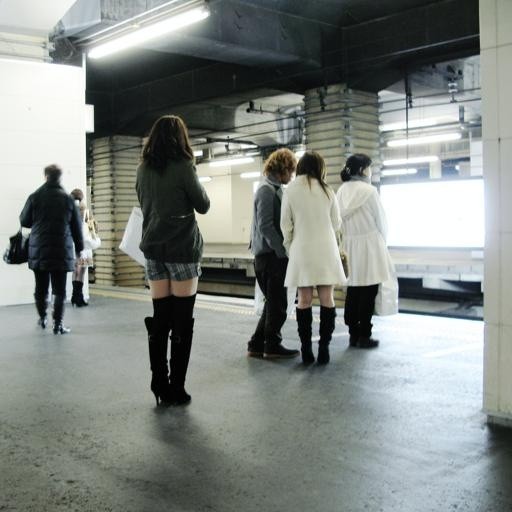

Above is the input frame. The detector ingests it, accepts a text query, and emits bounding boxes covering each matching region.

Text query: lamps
[383,154,439,167]
[378,113,461,133]
[240,170,261,179]
[385,130,463,148]
[381,168,418,177]
[209,154,255,168]
[198,176,212,184]
[85,2,213,62]
[192,149,205,158]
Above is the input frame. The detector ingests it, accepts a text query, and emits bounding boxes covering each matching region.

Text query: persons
[247,149,300,360]
[19,164,84,336]
[70,189,93,309]
[334,155,394,350]
[135,114,209,406]
[280,153,346,367]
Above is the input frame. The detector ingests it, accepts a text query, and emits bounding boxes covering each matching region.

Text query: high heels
[52,319,70,336]
[38,318,48,329]
[71,280,88,308]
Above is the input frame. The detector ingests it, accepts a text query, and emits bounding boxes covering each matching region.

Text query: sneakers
[264,345,299,358]
[359,339,379,348]
[349,336,359,347]
[247,343,263,357]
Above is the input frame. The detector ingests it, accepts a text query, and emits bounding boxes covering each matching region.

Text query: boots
[317,304,337,364]
[295,306,315,365]
[144,317,170,405]
[167,317,195,407]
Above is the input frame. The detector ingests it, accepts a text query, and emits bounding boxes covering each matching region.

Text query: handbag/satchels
[81,209,101,249]
[3,224,29,265]
[337,233,348,278]
[117,206,146,267]
[374,247,399,316]
[253,274,297,318]
